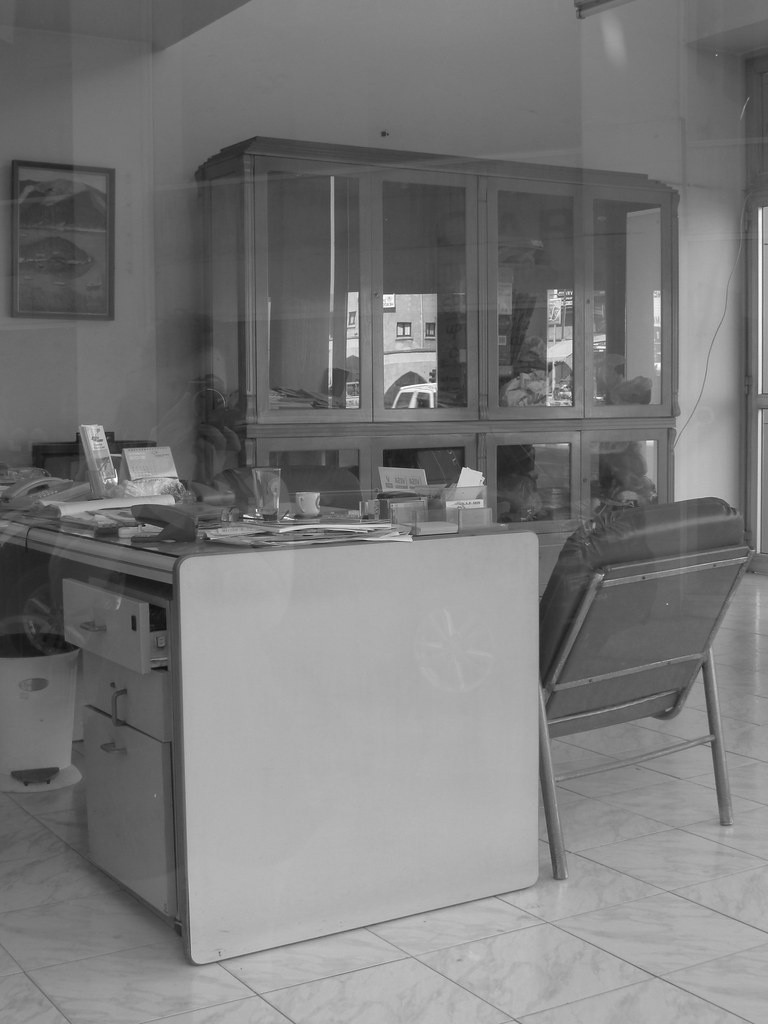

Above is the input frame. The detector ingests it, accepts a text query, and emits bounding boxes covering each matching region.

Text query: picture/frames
[11,159,115,320]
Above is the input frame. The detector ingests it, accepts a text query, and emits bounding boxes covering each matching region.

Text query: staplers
[131,505,198,542]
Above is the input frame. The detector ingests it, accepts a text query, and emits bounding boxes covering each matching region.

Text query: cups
[296,492,321,518]
[359,501,368,519]
[253,467,280,520]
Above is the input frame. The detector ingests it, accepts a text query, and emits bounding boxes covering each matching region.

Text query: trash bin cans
[0,633,82,793]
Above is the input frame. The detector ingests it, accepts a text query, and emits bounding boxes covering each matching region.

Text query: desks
[0,459,543,964]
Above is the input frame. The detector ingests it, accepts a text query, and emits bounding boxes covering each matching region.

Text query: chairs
[535,496,755,882]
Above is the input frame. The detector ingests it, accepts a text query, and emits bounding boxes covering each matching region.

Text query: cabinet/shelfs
[475,156,680,424]
[474,424,676,600]
[191,135,478,428]
[219,429,480,541]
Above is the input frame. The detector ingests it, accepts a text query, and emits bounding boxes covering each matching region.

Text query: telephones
[1,476,92,509]
